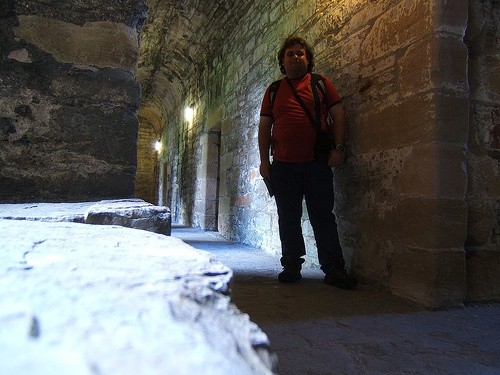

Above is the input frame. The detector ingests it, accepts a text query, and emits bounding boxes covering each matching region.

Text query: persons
[258,36,360,291]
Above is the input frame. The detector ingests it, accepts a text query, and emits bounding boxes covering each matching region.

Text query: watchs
[332,142,346,153]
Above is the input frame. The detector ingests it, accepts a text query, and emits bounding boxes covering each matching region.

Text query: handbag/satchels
[313,129,333,161]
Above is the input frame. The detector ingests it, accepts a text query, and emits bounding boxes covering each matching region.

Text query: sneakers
[324,269,357,289]
[279,268,301,282]
